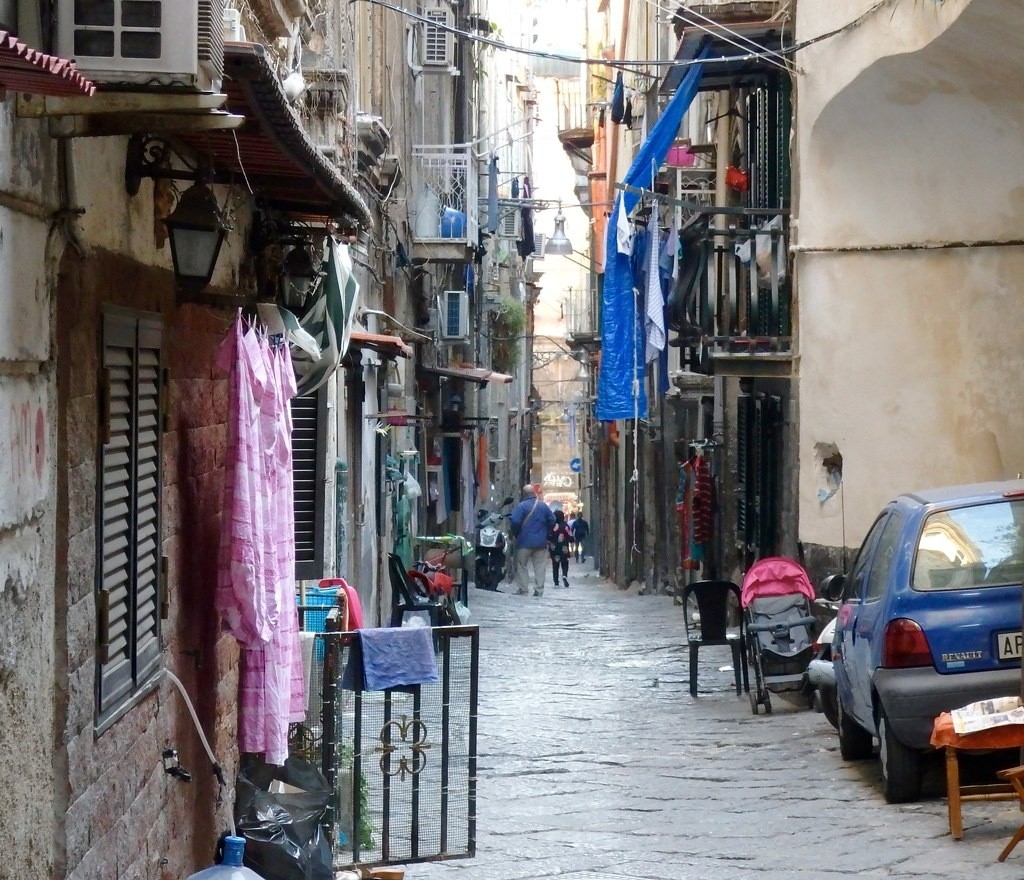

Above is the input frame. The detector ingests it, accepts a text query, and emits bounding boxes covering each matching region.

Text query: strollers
[737,556,826,715]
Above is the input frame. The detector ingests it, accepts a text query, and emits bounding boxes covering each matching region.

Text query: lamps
[248,227,322,296]
[127,133,272,289]
[450,395,461,411]
[478,196,573,257]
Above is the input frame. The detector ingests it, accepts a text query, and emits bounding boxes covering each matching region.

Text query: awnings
[125,42,376,239]
[656,20,792,95]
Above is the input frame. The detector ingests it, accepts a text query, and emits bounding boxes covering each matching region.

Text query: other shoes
[555,582,559,586]
[562,575,569,587]
[582,558,585,563]
[576,558,579,563]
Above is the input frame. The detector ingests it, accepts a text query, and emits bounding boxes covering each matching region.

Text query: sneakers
[512,588,528,596]
[532,589,543,597]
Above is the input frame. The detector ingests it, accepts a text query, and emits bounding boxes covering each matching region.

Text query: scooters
[475,497,516,590]
[807,598,840,729]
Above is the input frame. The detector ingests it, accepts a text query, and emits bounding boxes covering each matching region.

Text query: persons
[509,485,557,597]
[566,512,589,563]
[548,510,575,586]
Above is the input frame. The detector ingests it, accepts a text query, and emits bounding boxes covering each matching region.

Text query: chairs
[930,695,1024,841]
[386,553,449,654]
[998,765,1024,863]
[682,580,748,697]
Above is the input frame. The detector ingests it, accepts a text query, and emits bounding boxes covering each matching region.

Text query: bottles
[186,836,268,880]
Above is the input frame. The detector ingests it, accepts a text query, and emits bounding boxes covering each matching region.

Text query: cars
[821,478,1024,803]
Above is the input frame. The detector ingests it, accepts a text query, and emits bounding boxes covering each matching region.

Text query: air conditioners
[442,290,470,340]
[52,0,226,92]
[530,233,546,260]
[500,207,522,237]
[421,7,455,67]
